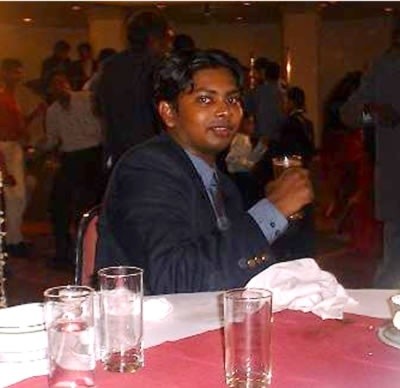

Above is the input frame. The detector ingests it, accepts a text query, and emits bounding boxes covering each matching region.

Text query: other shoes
[6,242,28,255]
[50,255,74,268]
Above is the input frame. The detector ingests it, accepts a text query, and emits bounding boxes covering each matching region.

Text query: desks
[1,288,400,388]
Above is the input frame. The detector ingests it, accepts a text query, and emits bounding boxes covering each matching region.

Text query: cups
[220,285,274,388]
[97,265,145,374]
[42,283,97,388]
[273,151,305,220]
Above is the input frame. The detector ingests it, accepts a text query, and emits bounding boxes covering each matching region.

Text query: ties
[209,172,227,229]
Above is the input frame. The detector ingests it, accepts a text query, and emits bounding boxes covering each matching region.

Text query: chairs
[0,142,26,249]
[73,208,99,292]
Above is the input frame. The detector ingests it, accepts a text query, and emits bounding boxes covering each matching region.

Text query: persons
[0,58,34,257]
[31,72,105,270]
[169,35,314,259]
[319,70,380,256]
[95,49,315,297]
[38,40,117,101]
[91,10,175,185]
[339,3,400,257]
